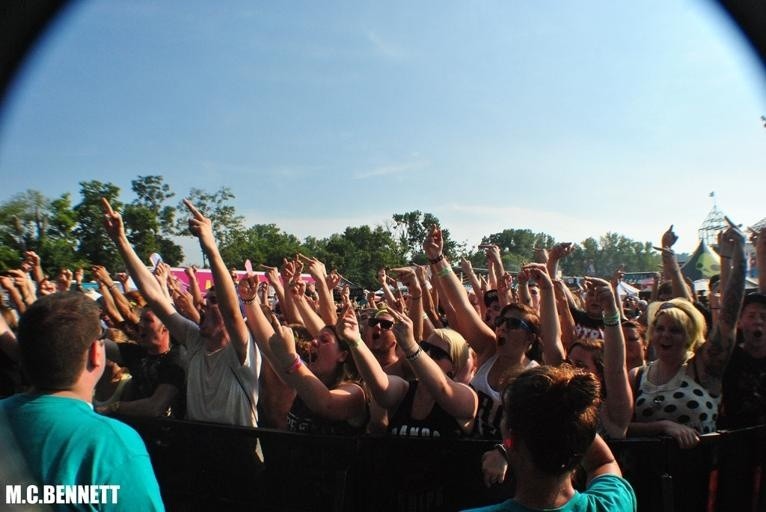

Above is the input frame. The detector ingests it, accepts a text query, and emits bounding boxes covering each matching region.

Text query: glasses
[88,327,109,352]
[367,318,394,329]
[419,340,454,363]
[494,315,533,334]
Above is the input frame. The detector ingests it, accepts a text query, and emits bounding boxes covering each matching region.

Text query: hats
[484,289,501,308]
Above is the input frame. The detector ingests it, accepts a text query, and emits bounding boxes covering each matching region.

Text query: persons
[2,192,766,511]
